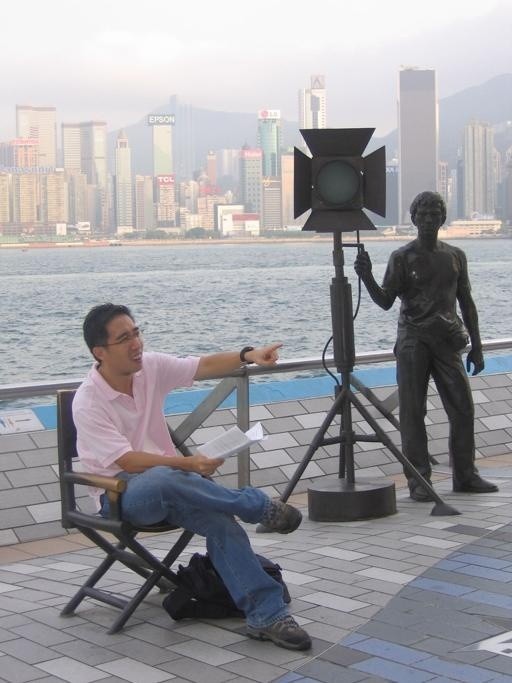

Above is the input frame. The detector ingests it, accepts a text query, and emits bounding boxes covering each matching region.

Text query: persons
[69,301,313,652]
[354,191,499,502]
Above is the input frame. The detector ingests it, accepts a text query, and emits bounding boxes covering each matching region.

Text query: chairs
[56,390,196,636]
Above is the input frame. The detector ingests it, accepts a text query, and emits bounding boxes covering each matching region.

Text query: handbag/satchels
[164,551,292,620]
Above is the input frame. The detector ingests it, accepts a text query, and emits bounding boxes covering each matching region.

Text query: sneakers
[245,615,311,651]
[259,499,303,535]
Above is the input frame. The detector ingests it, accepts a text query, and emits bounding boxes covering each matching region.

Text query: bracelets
[239,347,255,366]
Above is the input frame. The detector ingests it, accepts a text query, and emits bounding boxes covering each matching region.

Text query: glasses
[90,328,145,353]
